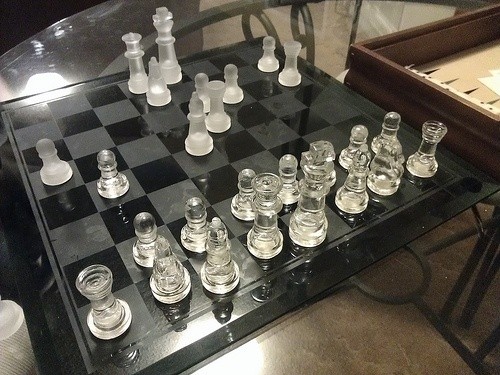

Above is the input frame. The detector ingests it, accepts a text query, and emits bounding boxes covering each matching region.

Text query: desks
[0,0,499,374]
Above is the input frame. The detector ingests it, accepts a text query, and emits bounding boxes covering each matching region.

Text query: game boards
[0,35,500,375]
